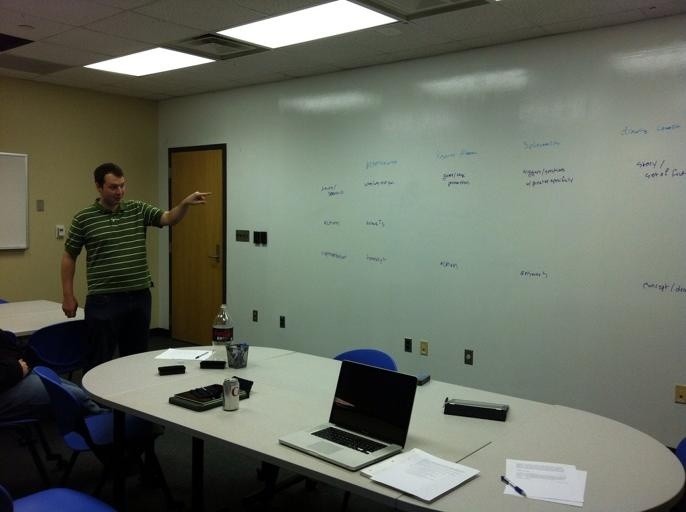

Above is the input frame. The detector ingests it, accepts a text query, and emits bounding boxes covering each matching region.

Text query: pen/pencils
[195,352,208,359]
[227,342,248,368]
[501,475,526,497]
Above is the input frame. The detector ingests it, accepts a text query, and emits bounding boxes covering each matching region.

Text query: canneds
[223,378,239,411]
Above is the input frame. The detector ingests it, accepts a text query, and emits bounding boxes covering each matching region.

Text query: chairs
[674,437,686,467]
[34,366,163,484]
[3,418,53,488]
[331,350,398,371]
[0,474,109,512]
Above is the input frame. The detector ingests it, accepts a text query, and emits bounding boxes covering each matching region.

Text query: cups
[226,343,248,369]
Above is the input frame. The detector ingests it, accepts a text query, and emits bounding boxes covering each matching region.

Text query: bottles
[212,304,234,368]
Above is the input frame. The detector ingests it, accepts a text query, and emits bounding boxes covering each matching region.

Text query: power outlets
[236,230,249,241]
[55,224,65,239]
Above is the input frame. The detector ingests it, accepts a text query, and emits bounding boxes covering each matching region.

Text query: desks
[1,300,86,340]
[81,343,686,511]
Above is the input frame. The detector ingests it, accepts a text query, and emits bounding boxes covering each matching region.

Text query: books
[360,447,480,503]
[169,383,249,412]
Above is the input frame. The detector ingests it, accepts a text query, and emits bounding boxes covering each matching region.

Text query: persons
[0,330,101,415]
[62,163,213,373]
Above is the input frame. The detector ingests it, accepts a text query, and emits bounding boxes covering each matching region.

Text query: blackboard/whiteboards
[0,152,30,250]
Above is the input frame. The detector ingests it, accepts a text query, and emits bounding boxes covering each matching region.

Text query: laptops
[278,359,418,472]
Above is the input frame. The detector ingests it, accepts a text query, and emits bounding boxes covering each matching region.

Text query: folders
[443,396,510,422]
